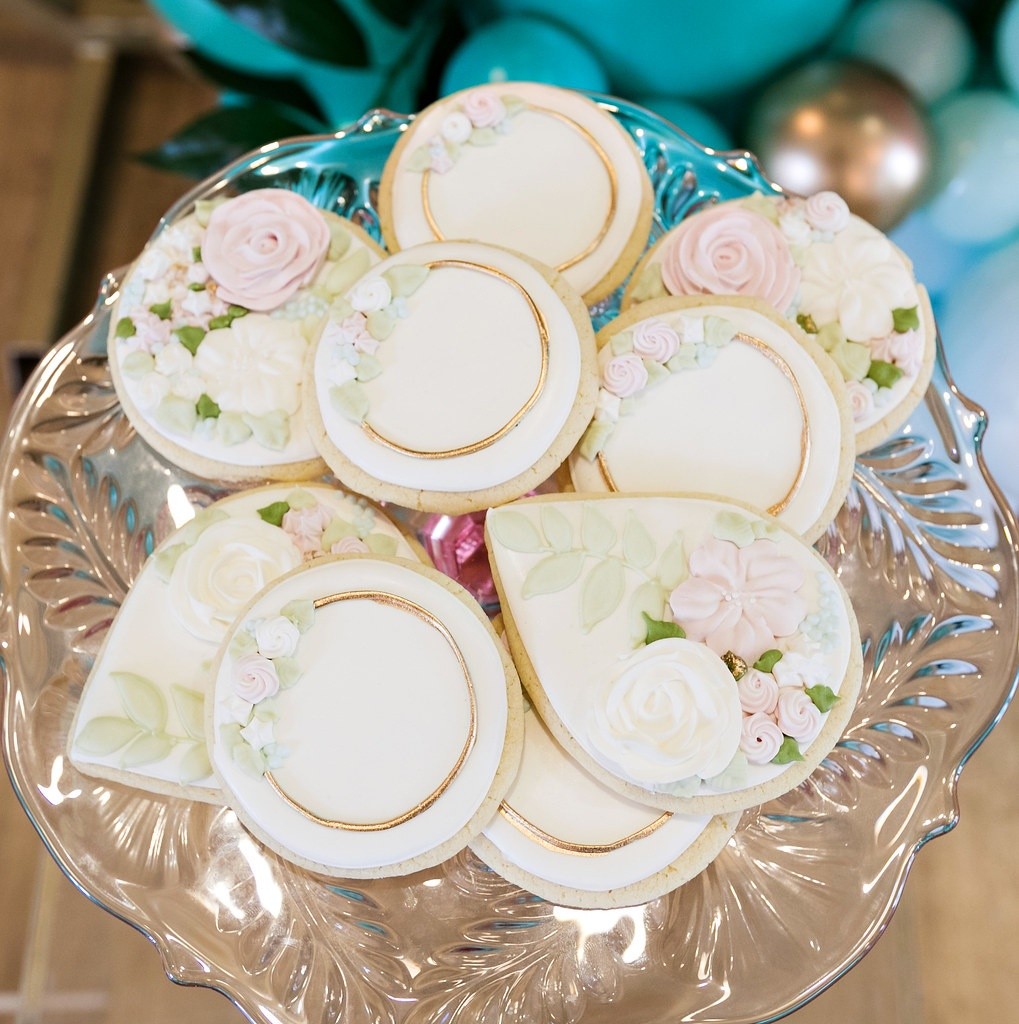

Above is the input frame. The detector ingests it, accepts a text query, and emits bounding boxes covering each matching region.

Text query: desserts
[62,80,939,917]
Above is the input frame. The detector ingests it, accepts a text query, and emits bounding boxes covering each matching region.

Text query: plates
[0,91,1019,1023]
